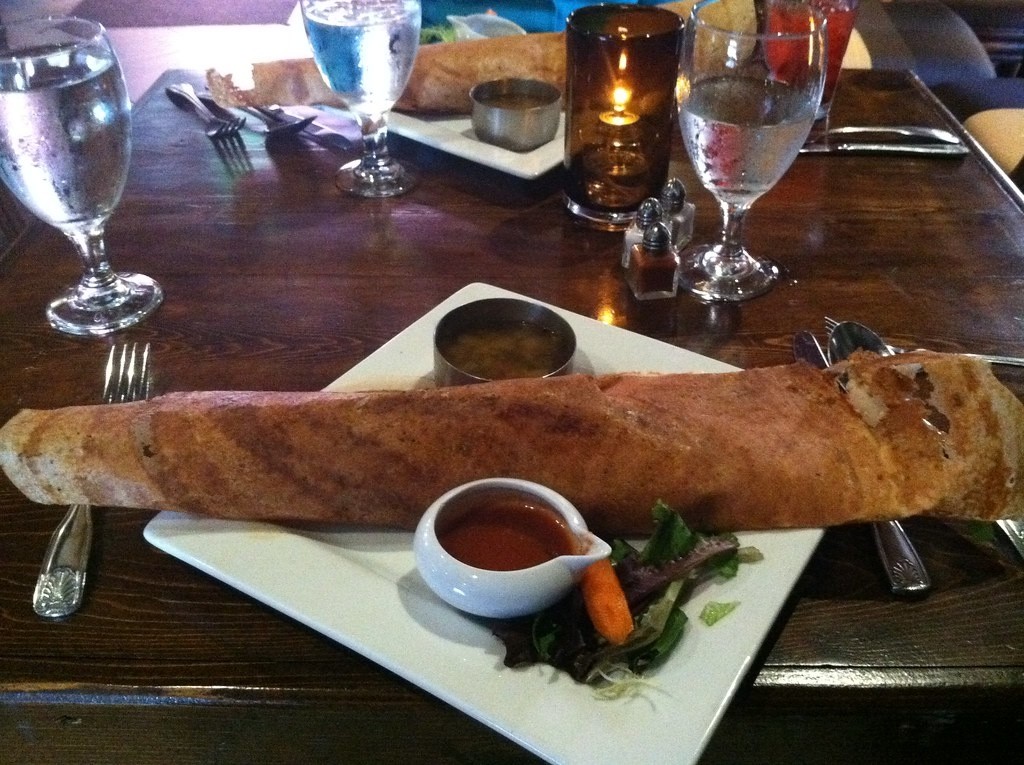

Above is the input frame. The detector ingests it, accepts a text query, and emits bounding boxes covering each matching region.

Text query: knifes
[793,142,971,154]
[794,327,931,597]
[258,101,353,152]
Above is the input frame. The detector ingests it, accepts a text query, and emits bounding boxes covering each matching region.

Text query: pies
[206,0,758,113]
[0,351,1024,529]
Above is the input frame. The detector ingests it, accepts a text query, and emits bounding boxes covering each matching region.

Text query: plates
[337,77,594,180]
[142,282,825,765]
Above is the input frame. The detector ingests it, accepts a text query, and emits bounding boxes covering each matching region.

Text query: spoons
[235,94,317,138]
[827,320,1024,572]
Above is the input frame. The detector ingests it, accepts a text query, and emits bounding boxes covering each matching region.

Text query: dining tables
[0,68,1024,765]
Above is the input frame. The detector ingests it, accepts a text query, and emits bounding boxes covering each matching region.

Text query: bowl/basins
[429,297,578,388]
[468,80,563,151]
[414,475,613,616]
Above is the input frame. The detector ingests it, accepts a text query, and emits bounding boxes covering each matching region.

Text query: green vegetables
[490,498,762,678]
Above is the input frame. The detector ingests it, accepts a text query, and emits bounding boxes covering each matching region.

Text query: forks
[820,315,1023,377]
[165,81,247,138]
[31,341,156,616]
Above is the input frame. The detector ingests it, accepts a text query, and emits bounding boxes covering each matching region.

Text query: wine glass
[0,13,166,337]
[300,1,422,196]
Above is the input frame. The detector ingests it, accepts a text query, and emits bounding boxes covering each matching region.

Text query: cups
[673,1,829,300]
[763,1,861,120]
[560,4,685,223]
